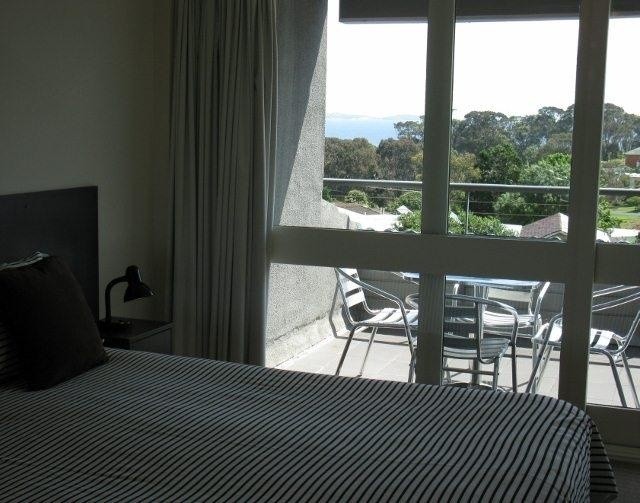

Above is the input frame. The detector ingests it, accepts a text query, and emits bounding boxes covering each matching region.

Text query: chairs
[329,266,418,377]
[405,292,518,393]
[526,285,639,408]
[451,277,552,393]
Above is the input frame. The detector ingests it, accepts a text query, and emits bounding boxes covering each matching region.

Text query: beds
[0,185,618,503]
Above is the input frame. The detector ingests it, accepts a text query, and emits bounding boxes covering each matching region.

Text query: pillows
[0,251,51,383]
[0,254,109,391]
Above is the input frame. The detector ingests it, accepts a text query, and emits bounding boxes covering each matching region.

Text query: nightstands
[99,317,175,355]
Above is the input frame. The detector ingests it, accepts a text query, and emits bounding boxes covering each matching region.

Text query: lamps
[100,264,161,331]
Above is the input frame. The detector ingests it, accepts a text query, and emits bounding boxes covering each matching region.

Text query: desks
[404,274,542,392]
